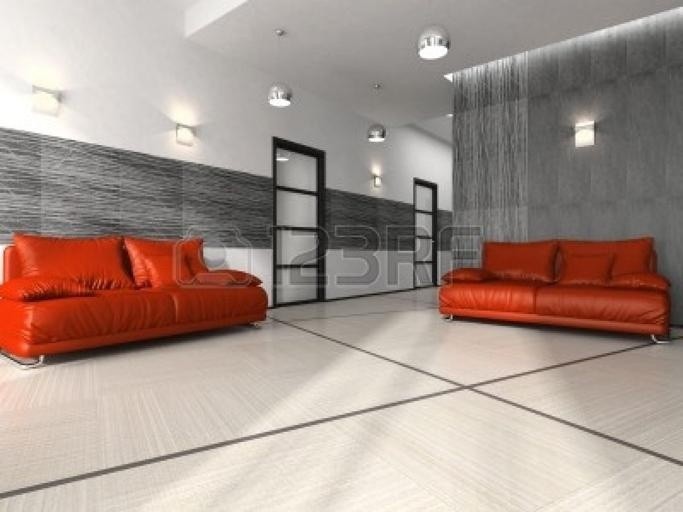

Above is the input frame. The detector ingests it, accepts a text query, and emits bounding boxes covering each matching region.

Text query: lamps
[364,123,386,144]
[266,81,292,108]
[413,23,450,60]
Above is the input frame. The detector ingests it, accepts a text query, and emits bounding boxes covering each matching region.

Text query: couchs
[0,231,267,368]
[437,236,673,344]
